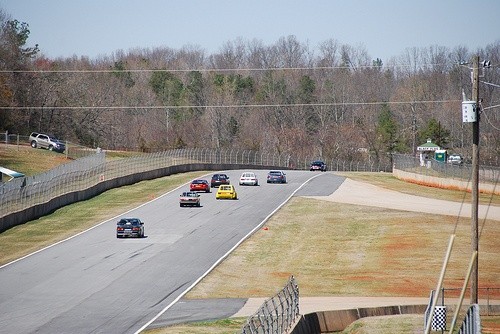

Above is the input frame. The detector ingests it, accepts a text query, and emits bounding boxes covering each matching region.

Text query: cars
[266,170,287,184]
[309,160,327,171]
[116,218,145,238]
[216,185,238,200]
[179,191,201,207]
[189,179,210,193]
[239,172,259,186]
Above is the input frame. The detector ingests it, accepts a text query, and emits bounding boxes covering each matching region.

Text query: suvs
[446,155,464,168]
[28,131,66,153]
[211,173,231,188]
